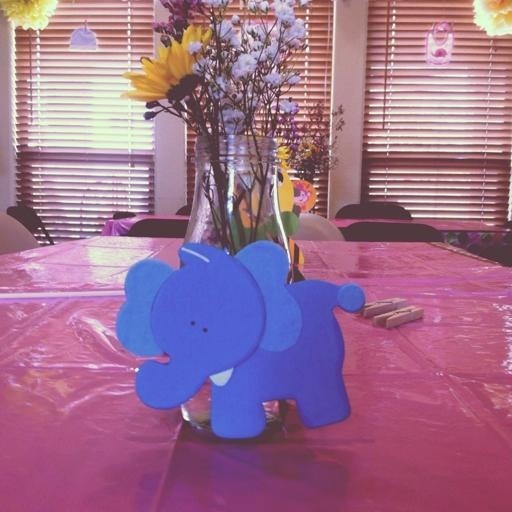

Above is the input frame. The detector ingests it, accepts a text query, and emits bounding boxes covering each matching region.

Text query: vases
[180,136,292,287]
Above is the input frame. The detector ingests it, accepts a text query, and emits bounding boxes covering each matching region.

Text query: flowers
[0,0,59,32]
[472,1,512,37]
[120,1,346,256]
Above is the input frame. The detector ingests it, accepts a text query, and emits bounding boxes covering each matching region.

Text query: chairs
[346,221,442,243]
[335,203,412,222]
[128,219,191,236]
[6,202,54,244]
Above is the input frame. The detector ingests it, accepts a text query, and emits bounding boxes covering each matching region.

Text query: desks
[104,214,511,237]
[1,237,511,511]
